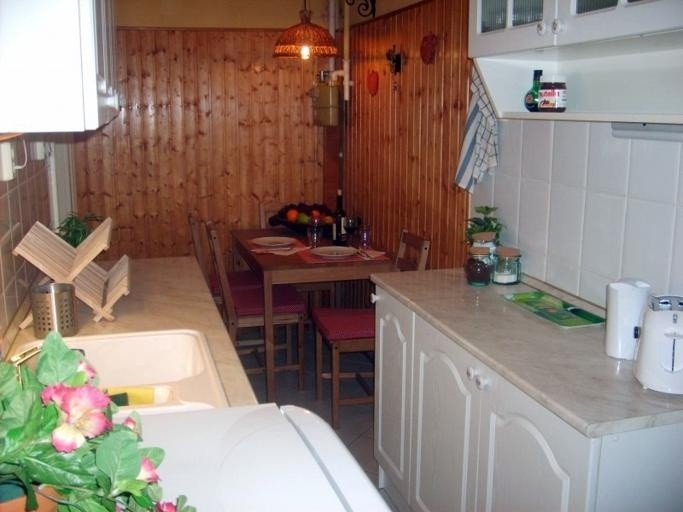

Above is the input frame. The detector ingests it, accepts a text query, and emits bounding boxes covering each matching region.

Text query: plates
[501,290,606,330]
[251,236,296,250]
[309,245,358,260]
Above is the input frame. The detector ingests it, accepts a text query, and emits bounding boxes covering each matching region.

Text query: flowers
[0,331,198,512]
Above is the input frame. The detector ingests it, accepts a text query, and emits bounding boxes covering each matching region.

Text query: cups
[359,224,371,250]
[343,216,358,244]
[307,217,321,247]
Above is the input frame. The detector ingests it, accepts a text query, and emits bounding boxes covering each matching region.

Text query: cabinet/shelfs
[0,0,119,133]
[0,255,259,408]
[370,268,683,509]
[466,0,683,57]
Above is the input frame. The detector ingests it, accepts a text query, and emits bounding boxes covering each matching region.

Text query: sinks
[5,329,230,423]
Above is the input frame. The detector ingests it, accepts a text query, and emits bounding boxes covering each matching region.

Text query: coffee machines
[604,278,652,360]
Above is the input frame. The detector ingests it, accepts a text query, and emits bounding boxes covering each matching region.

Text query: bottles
[524,70,542,112]
[466,248,491,287]
[538,75,567,113]
[492,247,521,284]
[332,189,346,243]
[471,232,496,265]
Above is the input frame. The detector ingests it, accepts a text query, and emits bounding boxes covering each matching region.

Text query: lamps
[273,2,338,57]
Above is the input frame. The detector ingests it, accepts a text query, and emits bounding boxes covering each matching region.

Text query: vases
[0,480,65,512]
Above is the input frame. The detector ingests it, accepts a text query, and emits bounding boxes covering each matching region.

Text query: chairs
[314,229,431,429]
[205,219,305,394]
[188,212,264,355]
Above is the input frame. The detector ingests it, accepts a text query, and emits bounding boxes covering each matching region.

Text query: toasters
[632,293,683,395]
